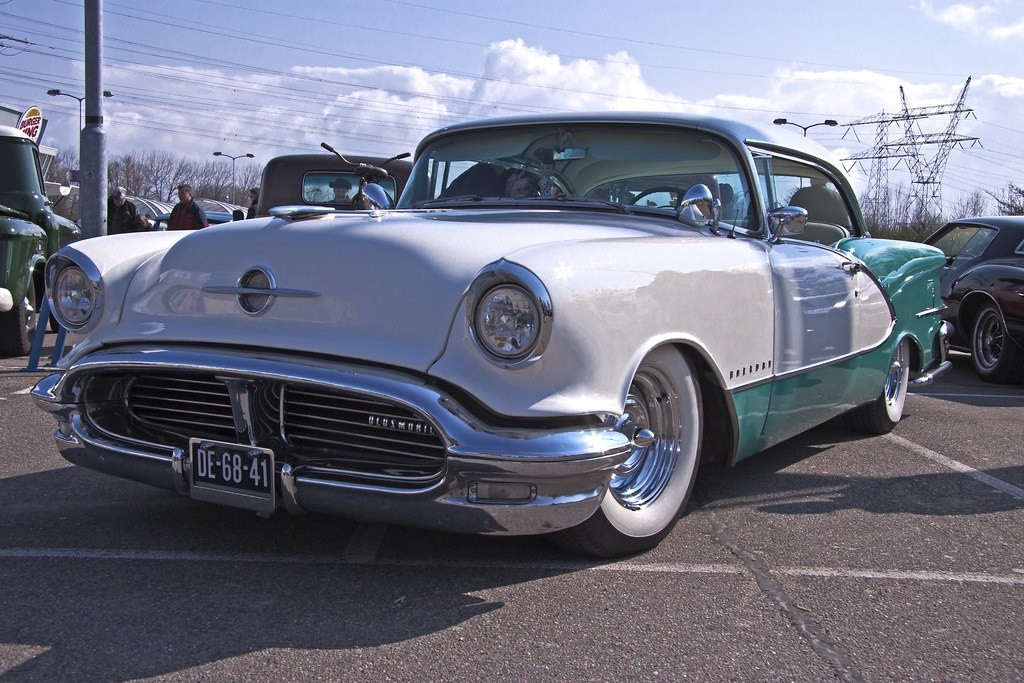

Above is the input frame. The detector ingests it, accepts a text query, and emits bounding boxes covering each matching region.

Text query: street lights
[48,87,115,132]
[772,117,838,188]
[211,151,255,205]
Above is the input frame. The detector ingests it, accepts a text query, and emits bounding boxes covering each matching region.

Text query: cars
[920,216,1024,383]
[194,198,250,222]
[253,154,431,219]
[126,195,176,232]
[0,123,60,357]
[28,106,947,557]
[53,211,82,249]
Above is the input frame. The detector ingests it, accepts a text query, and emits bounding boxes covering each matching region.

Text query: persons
[434,163,544,203]
[246,187,261,219]
[788,176,852,228]
[107,187,140,236]
[328,178,353,203]
[167,183,209,230]
[139,214,153,232]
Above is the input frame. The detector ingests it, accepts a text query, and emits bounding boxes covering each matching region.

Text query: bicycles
[320,140,410,210]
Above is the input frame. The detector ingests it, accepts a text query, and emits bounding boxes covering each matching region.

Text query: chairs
[719,183,851,246]
